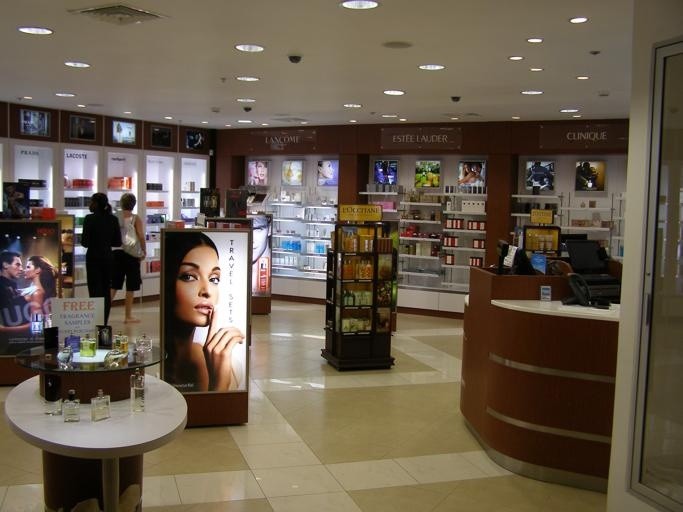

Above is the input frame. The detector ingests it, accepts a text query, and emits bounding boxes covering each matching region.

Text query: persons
[80,192,123,326]
[250,216,271,294]
[0,255,57,336]
[108,192,148,326]
[526,160,553,188]
[0,249,31,327]
[246,161,267,185]
[457,163,475,184]
[4,184,25,217]
[575,161,600,192]
[162,232,245,393]
[315,160,337,185]
[470,162,484,182]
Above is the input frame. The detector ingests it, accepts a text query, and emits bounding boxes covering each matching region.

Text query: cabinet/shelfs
[508,191,625,264]
[17,180,201,291]
[318,221,401,372]
[360,190,487,292]
[262,189,339,280]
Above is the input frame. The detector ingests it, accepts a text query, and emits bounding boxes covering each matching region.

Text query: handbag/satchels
[122,214,145,258]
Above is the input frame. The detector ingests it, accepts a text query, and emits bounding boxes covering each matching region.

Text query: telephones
[561,272,591,306]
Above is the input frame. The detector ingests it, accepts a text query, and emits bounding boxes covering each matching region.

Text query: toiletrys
[90,389,111,421]
[30,312,43,337]
[367,181,484,268]
[337,225,374,333]
[43,330,155,371]
[246,189,267,215]
[271,187,337,275]
[259,263,267,295]
[41,372,61,416]
[63,389,80,422]
[512,189,613,249]
[128,367,145,412]
[45,314,52,329]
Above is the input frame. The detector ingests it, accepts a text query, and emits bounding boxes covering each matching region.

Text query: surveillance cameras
[242,105,252,112]
[286,52,305,63]
[450,95,461,102]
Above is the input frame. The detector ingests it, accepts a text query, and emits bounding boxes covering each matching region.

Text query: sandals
[124,315,141,324]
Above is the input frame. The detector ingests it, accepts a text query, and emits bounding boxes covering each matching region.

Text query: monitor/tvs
[496,244,536,275]
[565,239,609,273]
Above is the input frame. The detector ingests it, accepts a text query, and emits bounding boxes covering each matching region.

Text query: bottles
[338,227,371,309]
[517,231,539,250]
[40,312,153,365]
[306,226,327,237]
[39,369,145,423]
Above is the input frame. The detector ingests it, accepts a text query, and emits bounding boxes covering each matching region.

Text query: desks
[3,341,190,511]
